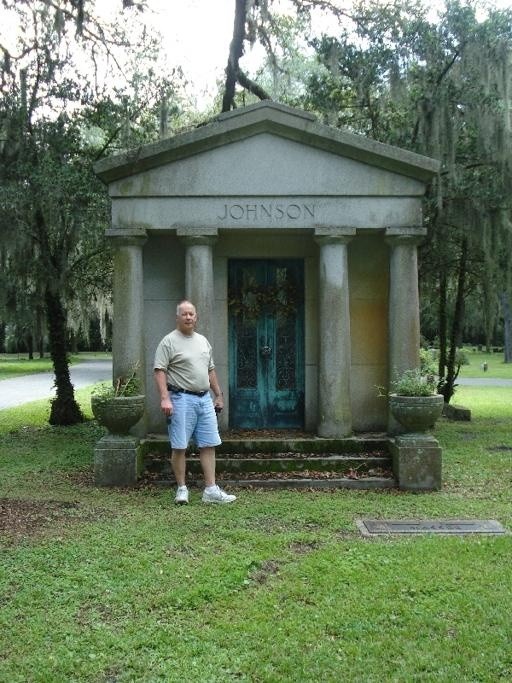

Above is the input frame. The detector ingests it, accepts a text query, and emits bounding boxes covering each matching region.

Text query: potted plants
[372,364,449,440]
[91,359,146,442]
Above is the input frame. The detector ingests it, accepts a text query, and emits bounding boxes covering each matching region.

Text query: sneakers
[174,486,189,504]
[202,485,237,503]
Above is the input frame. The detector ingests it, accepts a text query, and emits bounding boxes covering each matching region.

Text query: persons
[153,300,237,505]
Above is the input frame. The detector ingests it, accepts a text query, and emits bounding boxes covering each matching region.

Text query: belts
[168,384,208,396]
